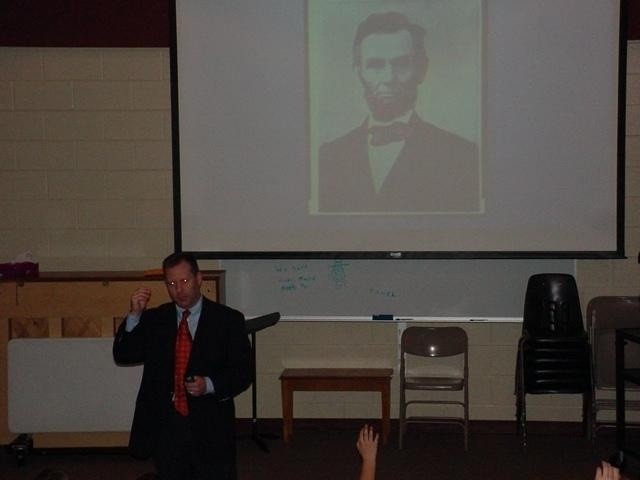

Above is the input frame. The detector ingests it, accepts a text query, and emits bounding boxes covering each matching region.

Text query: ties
[174,311,192,416]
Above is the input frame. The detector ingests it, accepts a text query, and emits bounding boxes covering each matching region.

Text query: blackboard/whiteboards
[216,257,578,325]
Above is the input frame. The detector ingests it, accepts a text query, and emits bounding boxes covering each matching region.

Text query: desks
[279,366,393,446]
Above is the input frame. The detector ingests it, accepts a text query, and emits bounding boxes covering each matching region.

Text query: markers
[469,319,488,321]
[395,318,414,320]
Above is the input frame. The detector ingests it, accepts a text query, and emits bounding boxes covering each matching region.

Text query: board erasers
[371,314,394,321]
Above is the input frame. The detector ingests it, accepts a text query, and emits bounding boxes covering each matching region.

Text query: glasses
[165,275,196,288]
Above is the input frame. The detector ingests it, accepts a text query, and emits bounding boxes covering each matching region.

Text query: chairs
[513,272,591,458]
[587,294,639,439]
[399,324,470,453]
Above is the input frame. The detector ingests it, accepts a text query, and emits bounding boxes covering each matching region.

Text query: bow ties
[369,122,408,145]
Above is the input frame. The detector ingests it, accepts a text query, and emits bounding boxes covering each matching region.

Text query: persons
[594,461,621,480]
[112,252,254,480]
[355,424,380,480]
[317,9,480,216]
